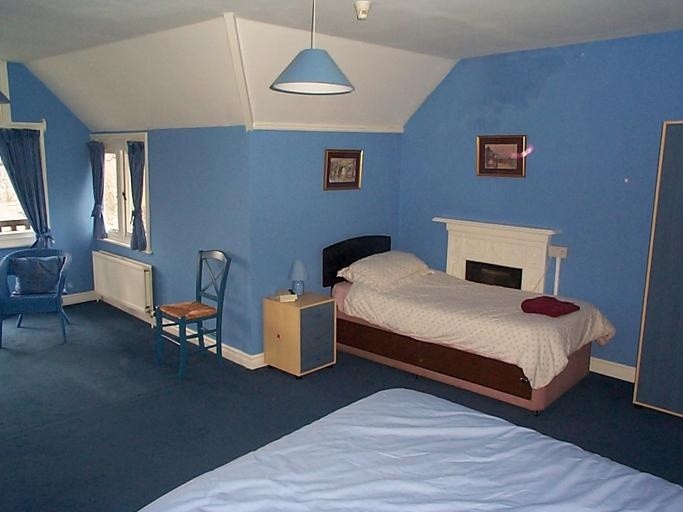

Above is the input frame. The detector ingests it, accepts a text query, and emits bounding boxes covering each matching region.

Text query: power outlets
[548,245,567,259]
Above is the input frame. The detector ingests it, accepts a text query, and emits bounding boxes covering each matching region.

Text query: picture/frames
[475,134,527,178]
[322,149,364,192]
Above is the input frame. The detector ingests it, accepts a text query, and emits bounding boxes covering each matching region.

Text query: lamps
[268,0,355,95]
[289,258,309,296]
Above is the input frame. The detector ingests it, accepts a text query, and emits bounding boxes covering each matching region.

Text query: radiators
[93,250,151,315]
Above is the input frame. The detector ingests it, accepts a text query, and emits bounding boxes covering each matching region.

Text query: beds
[322,234,616,415]
[138,387,682,512]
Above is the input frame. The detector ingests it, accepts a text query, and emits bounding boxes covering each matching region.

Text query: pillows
[11,256,68,295]
[337,250,436,291]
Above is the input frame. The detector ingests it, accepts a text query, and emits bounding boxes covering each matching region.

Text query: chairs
[1,247,73,348]
[157,250,231,384]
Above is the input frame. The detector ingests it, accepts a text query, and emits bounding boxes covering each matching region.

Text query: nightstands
[262,293,337,377]
